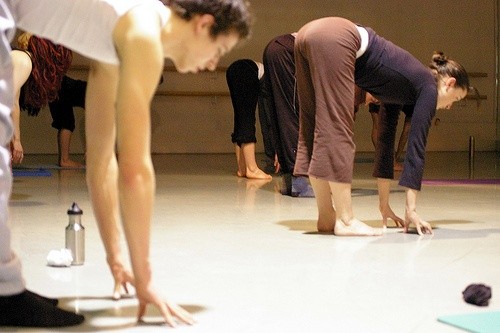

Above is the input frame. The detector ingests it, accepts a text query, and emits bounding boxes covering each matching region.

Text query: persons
[0,0,252,327]
[47,75,164,167]
[262,32,413,197]
[368,102,414,171]
[10,35,73,165]
[13,31,30,50]
[226,59,280,179]
[292,17,470,238]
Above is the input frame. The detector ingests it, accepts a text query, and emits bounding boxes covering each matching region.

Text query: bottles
[65,202,85,266]
